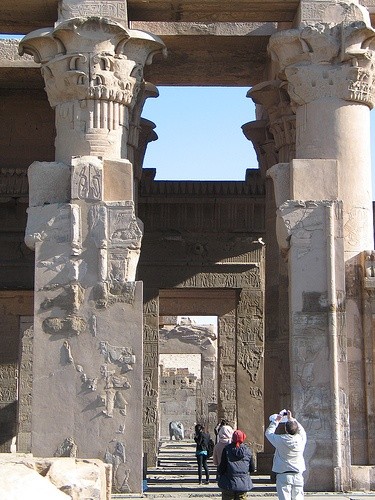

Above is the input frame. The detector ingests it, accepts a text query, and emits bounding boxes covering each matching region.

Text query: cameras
[282,412,288,415]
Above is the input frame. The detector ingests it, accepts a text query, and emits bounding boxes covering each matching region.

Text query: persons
[194,424,210,485]
[217,430,255,500]
[213,420,234,481]
[265,409,307,500]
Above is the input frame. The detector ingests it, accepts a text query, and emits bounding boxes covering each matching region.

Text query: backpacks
[201,432,214,457]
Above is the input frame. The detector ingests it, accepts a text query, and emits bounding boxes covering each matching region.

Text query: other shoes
[204,480,209,484]
[199,481,202,485]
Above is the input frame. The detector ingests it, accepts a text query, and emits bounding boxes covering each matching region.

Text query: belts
[276,471,298,474]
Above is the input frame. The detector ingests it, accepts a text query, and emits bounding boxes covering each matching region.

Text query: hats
[233,430,247,447]
